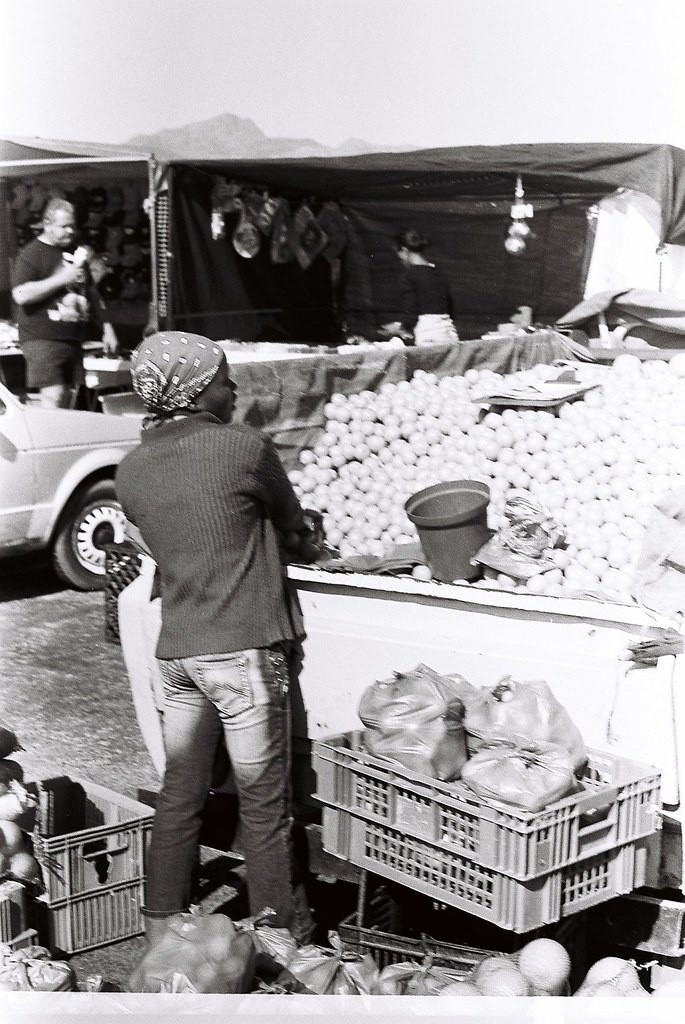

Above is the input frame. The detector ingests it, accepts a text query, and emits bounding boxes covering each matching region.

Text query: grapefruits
[437,938,646,996]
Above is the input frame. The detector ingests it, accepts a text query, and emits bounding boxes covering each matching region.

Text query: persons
[115,330,327,949]
[9,197,120,408]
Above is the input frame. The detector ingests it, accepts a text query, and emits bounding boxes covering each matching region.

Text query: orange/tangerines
[286,369,685,614]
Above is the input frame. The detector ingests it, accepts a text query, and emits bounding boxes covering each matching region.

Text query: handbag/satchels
[2,890,466,996]
[359,663,584,813]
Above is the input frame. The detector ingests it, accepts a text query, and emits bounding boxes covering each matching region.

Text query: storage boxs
[334,886,576,974]
[306,730,665,936]
[18,780,158,958]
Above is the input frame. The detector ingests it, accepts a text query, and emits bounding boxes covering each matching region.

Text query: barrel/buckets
[403,478,491,583]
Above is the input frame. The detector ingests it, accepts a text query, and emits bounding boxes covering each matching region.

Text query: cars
[0,378,148,592]
